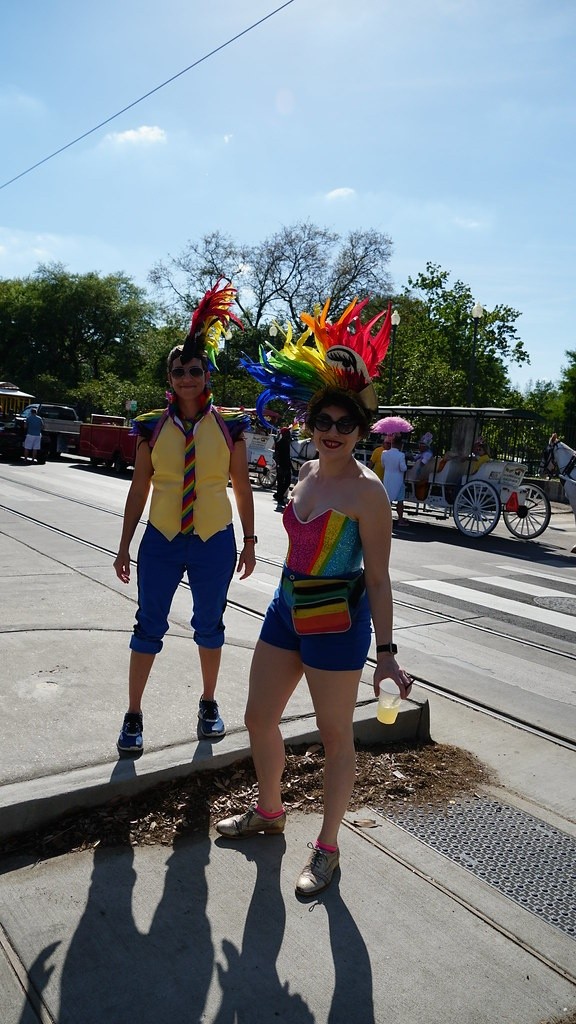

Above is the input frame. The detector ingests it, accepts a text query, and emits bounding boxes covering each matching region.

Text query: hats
[474,437,491,457]
[418,432,433,448]
[280,427,291,433]
[384,435,392,442]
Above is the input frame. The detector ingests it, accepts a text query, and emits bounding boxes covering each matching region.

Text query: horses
[537,433,576,553]
[289,436,319,476]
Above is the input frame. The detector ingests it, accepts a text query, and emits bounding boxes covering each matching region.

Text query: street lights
[466,301,484,409]
[220,328,234,407]
[387,309,402,405]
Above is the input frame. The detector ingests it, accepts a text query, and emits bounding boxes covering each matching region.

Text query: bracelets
[376,644,398,655]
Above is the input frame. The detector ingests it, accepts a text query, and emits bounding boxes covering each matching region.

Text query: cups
[376,678,402,724]
[126,400,137,411]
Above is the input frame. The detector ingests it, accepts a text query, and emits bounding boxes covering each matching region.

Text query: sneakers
[215,802,286,836]
[296,841,340,895]
[116,713,143,750]
[198,695,225,737]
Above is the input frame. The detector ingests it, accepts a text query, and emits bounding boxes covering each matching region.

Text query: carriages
[270,405,552,540]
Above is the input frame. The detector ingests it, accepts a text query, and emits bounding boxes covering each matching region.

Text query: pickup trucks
[15,402,84,432]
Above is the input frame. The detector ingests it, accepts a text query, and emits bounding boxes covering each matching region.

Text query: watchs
[244,536,258,544]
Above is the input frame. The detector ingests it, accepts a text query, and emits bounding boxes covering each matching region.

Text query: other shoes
[397,521,409,526]
[272,493,286,506]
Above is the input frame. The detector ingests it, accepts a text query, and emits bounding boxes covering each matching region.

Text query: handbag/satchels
[292,580,356,633]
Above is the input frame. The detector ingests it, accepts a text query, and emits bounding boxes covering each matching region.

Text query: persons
[381,437,409,526]
[273,426,292,511]
[111,342,258,752]
[214,389,414,899]
[129,413,140,435]
[367,436,392,484]
[470,442,491,476]
[409,440,433,471]
[22,409,45,463]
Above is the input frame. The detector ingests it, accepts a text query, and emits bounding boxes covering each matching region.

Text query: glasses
[171,366,207,379]
[311,411,359,434]
[477,448,484,452]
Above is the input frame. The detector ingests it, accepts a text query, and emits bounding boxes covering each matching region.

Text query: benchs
[429,460,466,483]
[462,461,528,488]
[405,458,436,481]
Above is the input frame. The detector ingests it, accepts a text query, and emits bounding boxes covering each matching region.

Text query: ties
[170,412,205,534]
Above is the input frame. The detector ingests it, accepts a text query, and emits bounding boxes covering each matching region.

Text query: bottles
[125,410,137,427]
